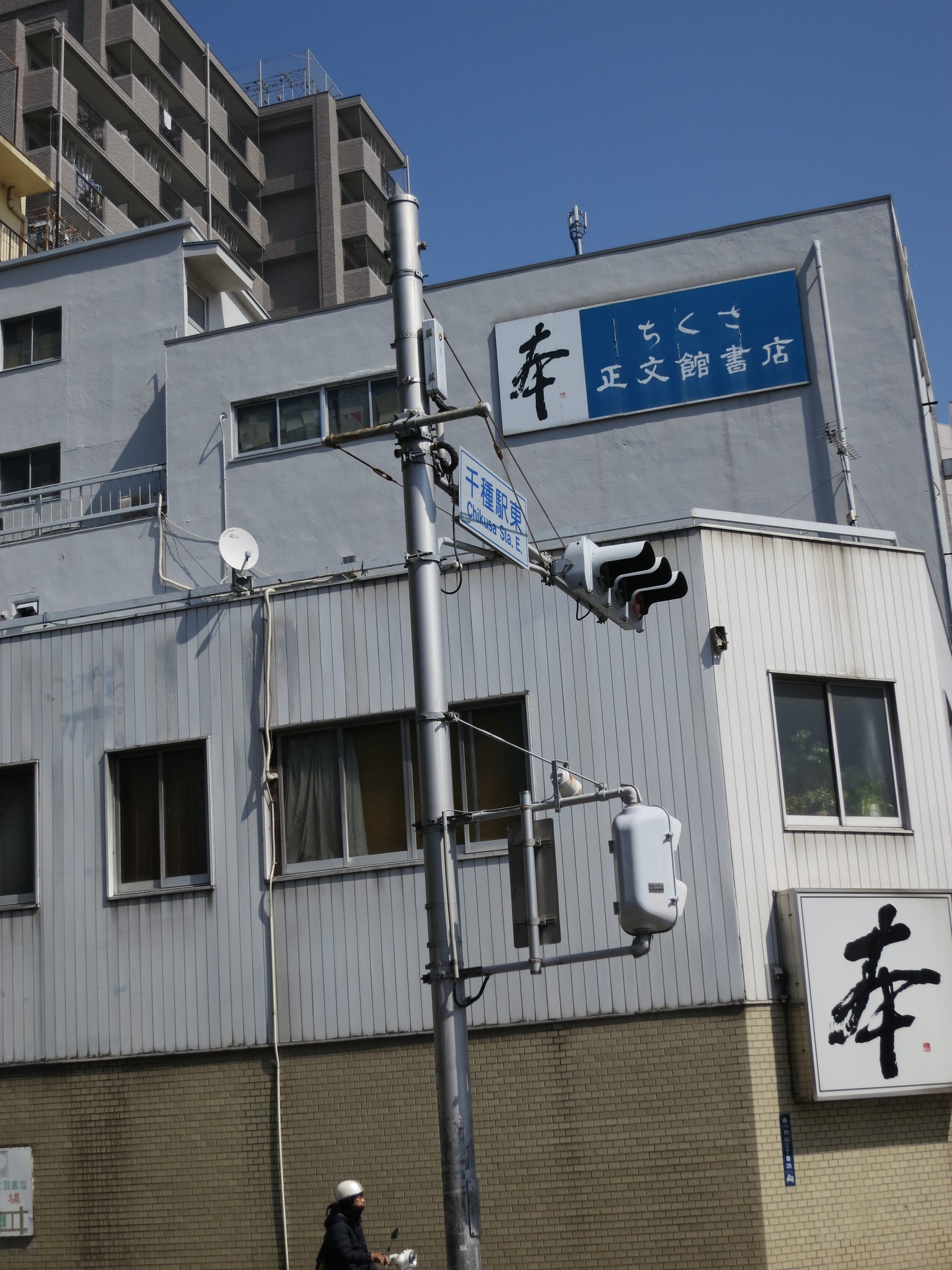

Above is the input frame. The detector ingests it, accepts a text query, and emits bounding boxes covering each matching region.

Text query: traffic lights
[611,804,688,936]
[561,535,689,634]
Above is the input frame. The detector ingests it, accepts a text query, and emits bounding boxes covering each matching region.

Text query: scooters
[372,1228,418,1270]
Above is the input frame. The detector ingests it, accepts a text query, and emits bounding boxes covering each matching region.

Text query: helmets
[336,1179,364,1201]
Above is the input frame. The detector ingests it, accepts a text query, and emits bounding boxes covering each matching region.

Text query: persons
[316,1181,391,1270]
